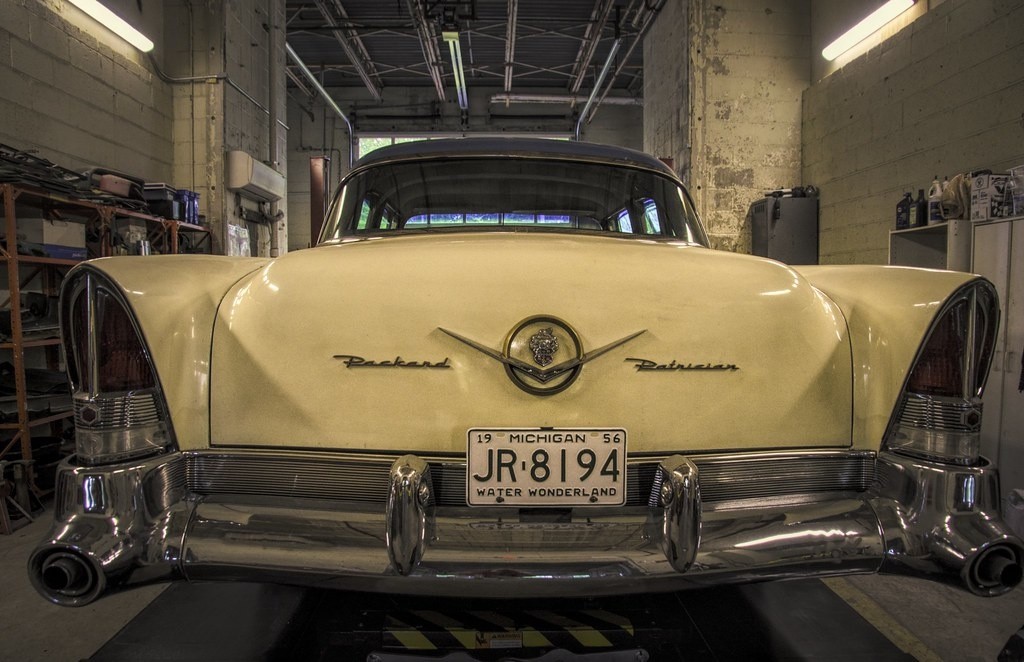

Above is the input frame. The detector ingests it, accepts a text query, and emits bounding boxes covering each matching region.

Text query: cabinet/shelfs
[0,184,106,539]
[106,206,214,256]
[751,196,819,266]
[887,220,1024,500]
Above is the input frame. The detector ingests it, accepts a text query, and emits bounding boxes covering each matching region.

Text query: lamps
[67,0,155,53]
[442,6,461,41]
[820,0,917,62]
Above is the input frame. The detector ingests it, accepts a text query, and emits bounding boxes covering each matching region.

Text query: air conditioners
[228,150,285,203]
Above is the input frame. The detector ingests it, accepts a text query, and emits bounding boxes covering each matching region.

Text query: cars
[23,135,1024,609]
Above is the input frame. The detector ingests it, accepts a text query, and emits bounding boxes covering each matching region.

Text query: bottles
[896,176,949,230]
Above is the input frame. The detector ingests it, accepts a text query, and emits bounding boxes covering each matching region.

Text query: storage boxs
[145,183,201,223]
[0,218,86,258]
[970,174,1024,223]
